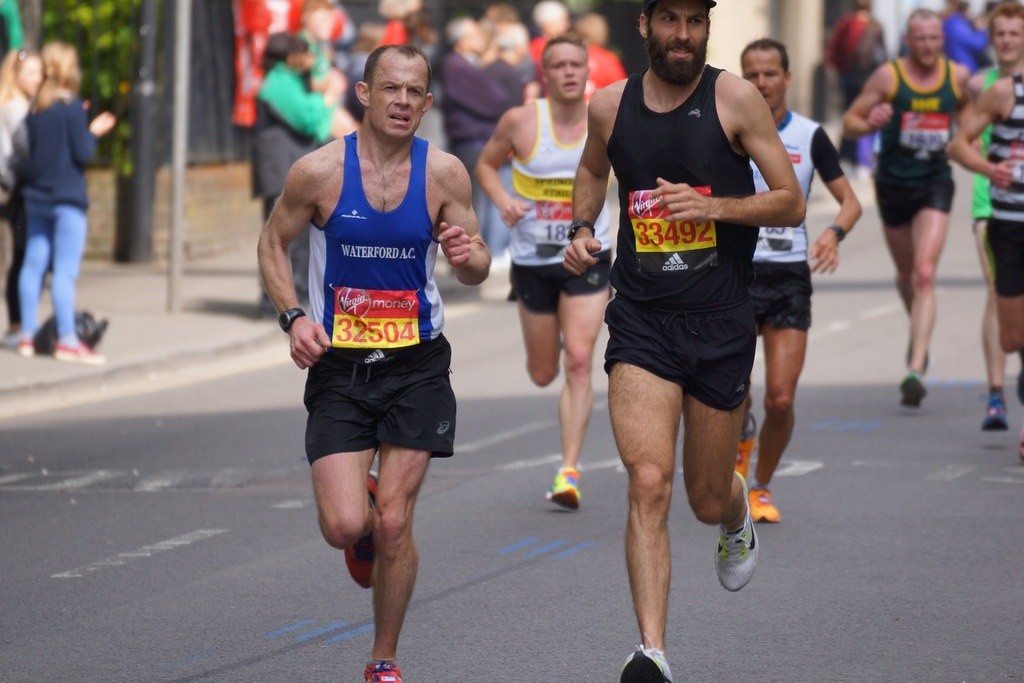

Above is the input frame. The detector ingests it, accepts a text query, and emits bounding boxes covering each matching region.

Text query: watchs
[278,308,305,333]
[567,221,596,241]
[827,225,844,240]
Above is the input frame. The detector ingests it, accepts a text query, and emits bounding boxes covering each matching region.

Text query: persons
[0,40,112,366]
[827,0,1024,458]
[708,40,863,525]
[478,32,613,510]
[256,44,491,683]
[250,0,626,320]
[545,0,806,683]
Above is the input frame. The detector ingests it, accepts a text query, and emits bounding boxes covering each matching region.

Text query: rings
[675,203,679,210]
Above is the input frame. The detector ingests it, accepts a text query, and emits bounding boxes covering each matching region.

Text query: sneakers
[620,633,674,683]
[747,487,780,523]
[55,341,105,366]
[17,340,34,356]
[715,470,758,593]
[364,663,404,683]
[343,471,380,589]
[898,373,924,407]
[546,468,581,509]
[733,414,756,482]
[981,401,1008,429]
